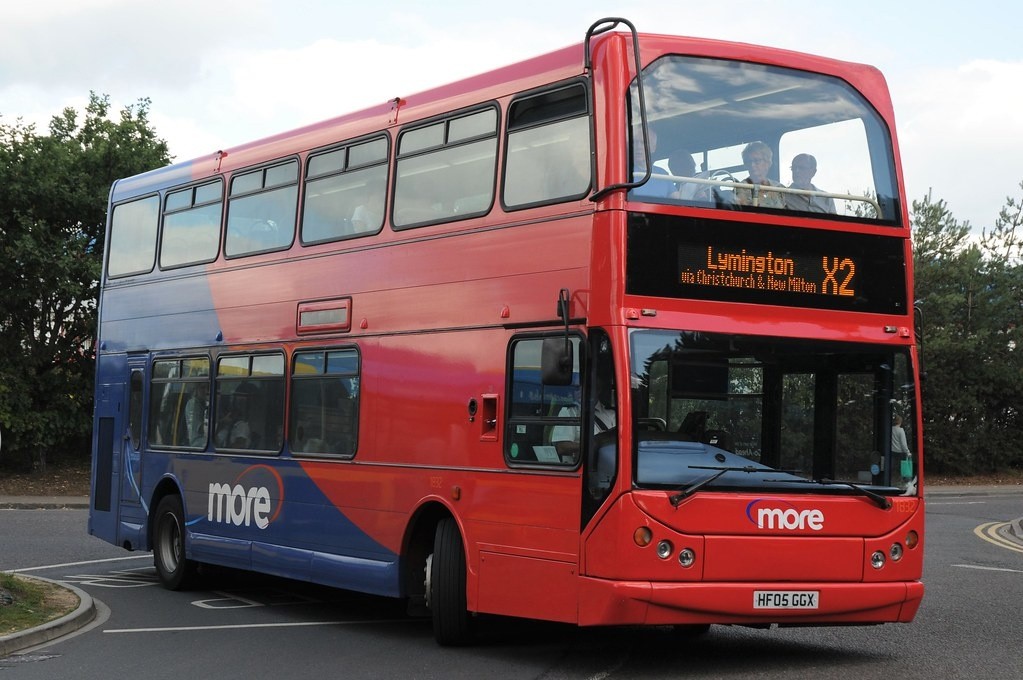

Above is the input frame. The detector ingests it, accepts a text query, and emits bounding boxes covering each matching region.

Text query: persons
[732,142,787,208]
[185,374,355,455]
[351,179,387,234]
[551,373,617,467]
[632,127,708,201]
[785,153,837,214]
[669,150,721,201]
[892,416,912,483]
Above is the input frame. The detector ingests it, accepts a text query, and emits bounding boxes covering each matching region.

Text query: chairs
[231,170,738,255]
[139,367,583,466]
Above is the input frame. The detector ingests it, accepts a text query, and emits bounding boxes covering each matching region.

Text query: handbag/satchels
[900,455,914,478]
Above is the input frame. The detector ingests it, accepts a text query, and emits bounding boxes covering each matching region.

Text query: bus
[87,17,926,650]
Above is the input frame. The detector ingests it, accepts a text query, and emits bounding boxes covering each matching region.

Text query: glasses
[790,166,810,171]
[743,157,764,165]
